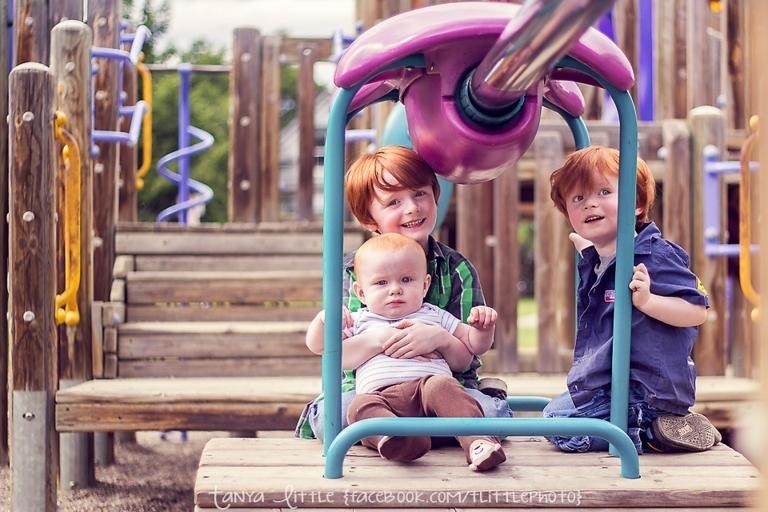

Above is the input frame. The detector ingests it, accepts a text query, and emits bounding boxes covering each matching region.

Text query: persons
[294,145,515,440]
[541,145,722,452]
[303,231,508,473]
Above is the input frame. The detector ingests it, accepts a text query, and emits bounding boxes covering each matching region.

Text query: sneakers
[377,436,433,464]
[468,437,508,472]
[642,409,722,453]
